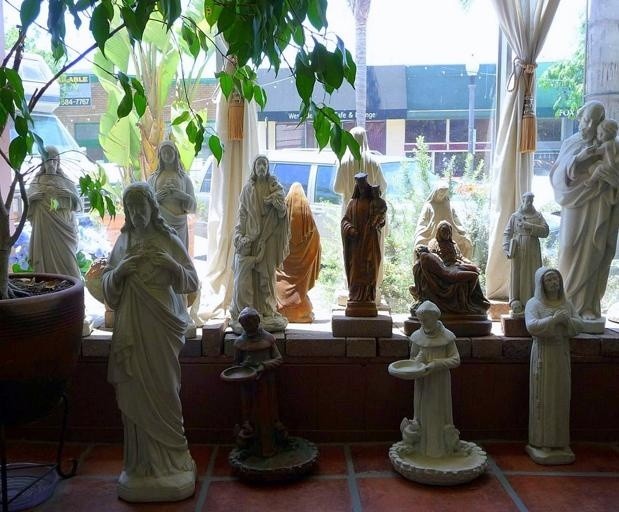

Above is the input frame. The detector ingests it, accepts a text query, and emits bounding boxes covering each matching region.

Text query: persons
[408,297,466,453]
[228,154,291,317]
[273,179,325,321]
[20,143,94,319]
[404,217,492,315]
[544,98,617,321]
[332,121,389,291]
[144,140,197,307]
[228,303,286,459]
[498,192,555,322]
[409,183,475,260]
[99,179,202,476]
[586,113,618,189]
[337,169,389,317]
[522,265,587,450]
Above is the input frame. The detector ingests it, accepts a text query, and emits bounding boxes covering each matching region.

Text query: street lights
[462,51,481,176]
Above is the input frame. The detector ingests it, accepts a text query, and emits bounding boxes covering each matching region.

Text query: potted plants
[0,0,367,425]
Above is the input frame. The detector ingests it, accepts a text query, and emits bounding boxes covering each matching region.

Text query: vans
[189,146,427,260]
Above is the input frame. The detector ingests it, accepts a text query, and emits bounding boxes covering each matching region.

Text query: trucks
[3,48,106,223]
